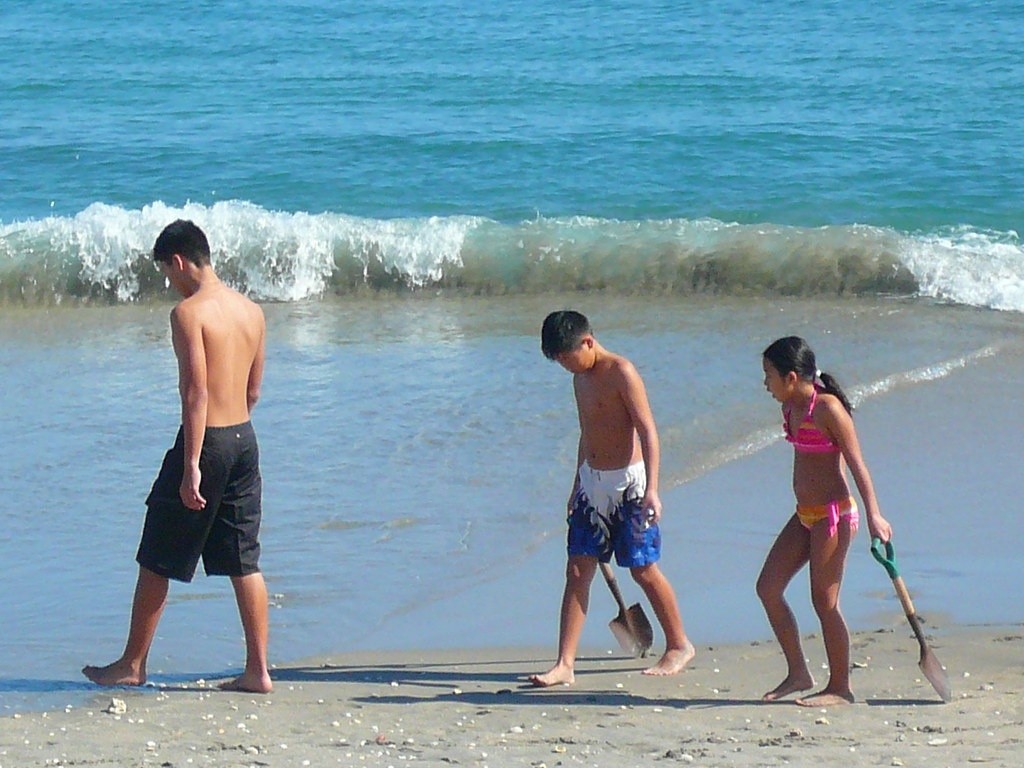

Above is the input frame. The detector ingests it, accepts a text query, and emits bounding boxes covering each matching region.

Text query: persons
[526,310,697,686]
[752,336,893,707]
[81,218,274,696]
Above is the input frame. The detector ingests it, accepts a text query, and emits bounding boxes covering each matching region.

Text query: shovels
[868,531,954,705]
[595,558,654,660]
